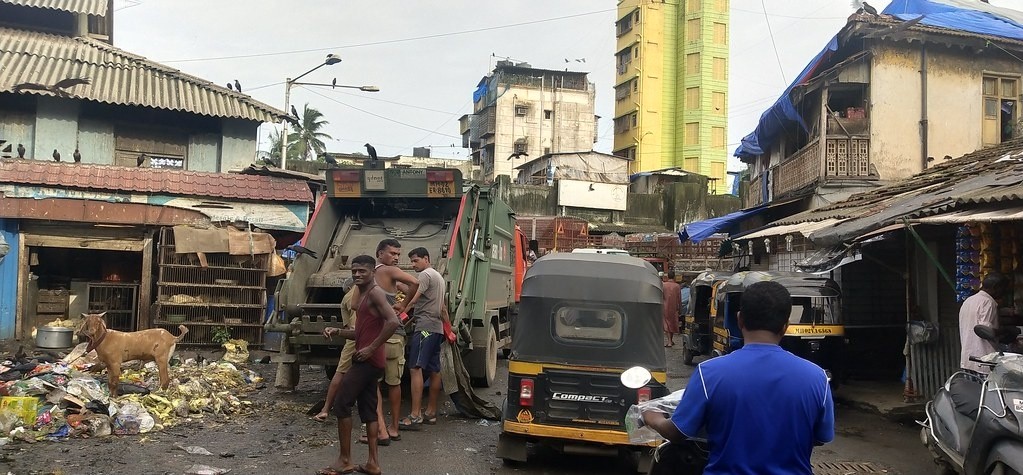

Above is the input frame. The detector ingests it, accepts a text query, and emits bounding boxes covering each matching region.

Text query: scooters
[914,326,1023,475]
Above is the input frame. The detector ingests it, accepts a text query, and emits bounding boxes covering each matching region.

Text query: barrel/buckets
[262,293,292,353]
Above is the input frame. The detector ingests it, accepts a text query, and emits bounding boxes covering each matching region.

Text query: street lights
[280,55,380,170]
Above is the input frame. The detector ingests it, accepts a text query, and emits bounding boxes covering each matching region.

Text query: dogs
[80,311,189,398]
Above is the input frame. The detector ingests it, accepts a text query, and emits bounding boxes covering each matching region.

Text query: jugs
[106,273,121,281]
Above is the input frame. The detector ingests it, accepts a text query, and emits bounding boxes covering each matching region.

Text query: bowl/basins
[36,328,74,348]
[224,317,242,323]
[166,313,186,321]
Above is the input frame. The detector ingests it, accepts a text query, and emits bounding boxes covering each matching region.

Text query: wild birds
[17,143,82,162]
[332,77,336,89]
[226,79,241,92]
[492,51,587,73]
[364,143,378,160]
[13,78,92,97]
[137,153,146,167]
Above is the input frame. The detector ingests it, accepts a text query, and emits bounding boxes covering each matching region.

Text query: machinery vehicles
[263,157,538,390]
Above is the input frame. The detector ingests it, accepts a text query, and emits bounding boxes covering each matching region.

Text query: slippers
[672,342,675,346]
[359,435,390,446]
[313,413,324,422]
[387,428,402,441]
[315,465,354,475]
[355,466,381,475]
[664,343,672,347]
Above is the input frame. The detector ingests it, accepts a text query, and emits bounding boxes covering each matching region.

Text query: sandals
[399,415,423,431]
[421,412,436,425]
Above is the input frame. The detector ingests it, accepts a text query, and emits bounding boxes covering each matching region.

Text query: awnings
[677,197,805,244]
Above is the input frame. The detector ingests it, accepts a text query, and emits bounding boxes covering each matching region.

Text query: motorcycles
[712,271,849,392]
[683,269,745,366]
[492,251,670,475]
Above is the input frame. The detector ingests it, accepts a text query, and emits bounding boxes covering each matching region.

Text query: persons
[663,271,682,347]
[642,282,834,475]
[959,272,1010,384]
[313,239,445,475]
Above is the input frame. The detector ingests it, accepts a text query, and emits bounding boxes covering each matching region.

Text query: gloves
[443,320,456,344]
